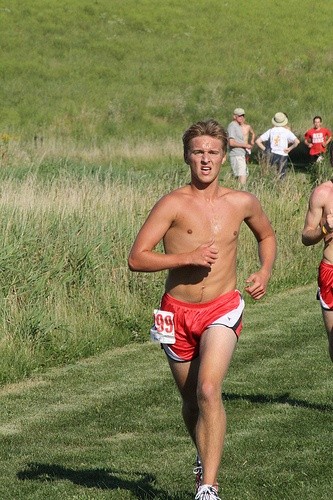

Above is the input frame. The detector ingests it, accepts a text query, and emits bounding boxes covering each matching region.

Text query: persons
[304,116,332,162]
[255,112,300,179]
[227,108,255,184]
[302,179,333,364]
[128,121,277,500]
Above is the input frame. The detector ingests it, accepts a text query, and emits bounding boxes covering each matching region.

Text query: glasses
[237,114,244,117]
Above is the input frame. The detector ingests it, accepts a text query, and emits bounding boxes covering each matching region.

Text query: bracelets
[321,225,328,235]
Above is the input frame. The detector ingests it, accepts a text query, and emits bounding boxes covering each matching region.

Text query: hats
[233,108,245,115]
[271,112,288,127]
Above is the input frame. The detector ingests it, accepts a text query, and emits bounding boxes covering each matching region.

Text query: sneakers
[191,454,218,500]
